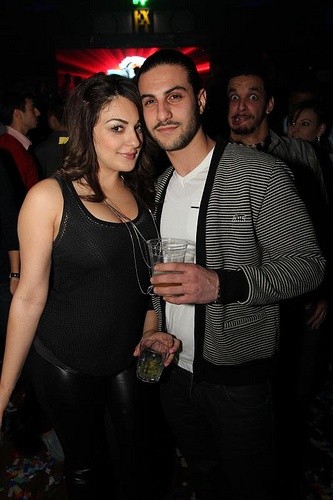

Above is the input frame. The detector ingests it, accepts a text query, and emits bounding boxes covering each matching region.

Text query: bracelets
[207,269,221,305]
[9,273,20,278]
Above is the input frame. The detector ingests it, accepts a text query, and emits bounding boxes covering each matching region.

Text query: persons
[135,47,326,500]
[0,67,139,375]
[222,69,333,458]
[0,73,181,500]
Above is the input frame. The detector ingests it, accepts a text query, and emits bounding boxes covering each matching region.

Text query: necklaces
[230,137,265,146]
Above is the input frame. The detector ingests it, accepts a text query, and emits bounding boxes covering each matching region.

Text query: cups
[149,237,189,298]
[135,338,170,383]
